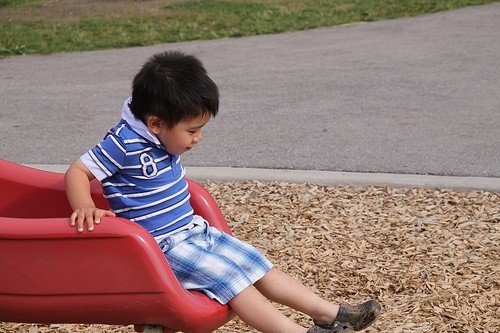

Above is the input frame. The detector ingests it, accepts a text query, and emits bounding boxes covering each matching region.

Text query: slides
[0,160,237,333]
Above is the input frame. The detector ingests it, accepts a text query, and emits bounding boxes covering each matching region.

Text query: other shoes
[306,321,355,333]
[313,300,381,331]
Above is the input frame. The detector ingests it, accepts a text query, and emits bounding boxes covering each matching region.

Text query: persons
[64,49,381,333]
